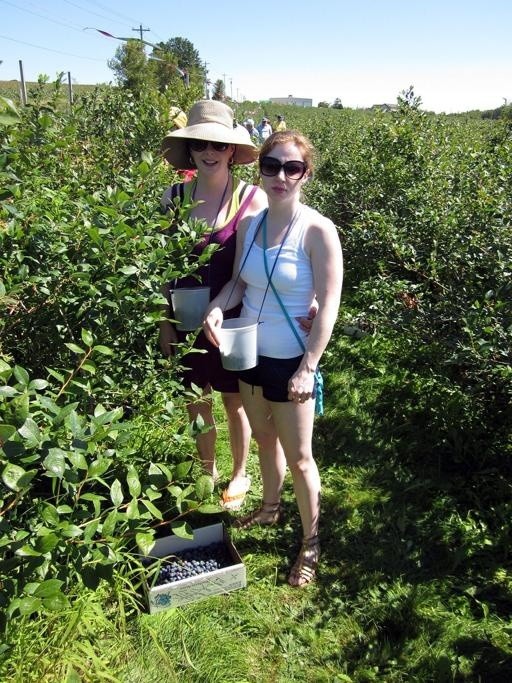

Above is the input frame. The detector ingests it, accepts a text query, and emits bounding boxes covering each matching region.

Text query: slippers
[217,477,249,512]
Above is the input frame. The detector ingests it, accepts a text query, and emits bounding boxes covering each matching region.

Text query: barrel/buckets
[215,317,262,372]
[170,286,212,333]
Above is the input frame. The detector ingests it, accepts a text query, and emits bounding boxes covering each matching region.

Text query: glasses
[258,156,308,182]
[189,139,230,153]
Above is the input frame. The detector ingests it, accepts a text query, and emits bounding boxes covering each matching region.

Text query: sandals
[288,534,322,587]
[236,502,282,529]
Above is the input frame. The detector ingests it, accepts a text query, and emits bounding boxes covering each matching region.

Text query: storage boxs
[136,512,248,614]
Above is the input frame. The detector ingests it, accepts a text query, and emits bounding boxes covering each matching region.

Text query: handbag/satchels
[314,366,325,416]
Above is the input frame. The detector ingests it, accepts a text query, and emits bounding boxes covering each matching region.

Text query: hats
[262,118,267,121]
[166,106,187,130]
[161,100,259,171]
[243,119,255,125]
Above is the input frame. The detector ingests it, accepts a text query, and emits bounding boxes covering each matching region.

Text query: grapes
[143,544,231,584]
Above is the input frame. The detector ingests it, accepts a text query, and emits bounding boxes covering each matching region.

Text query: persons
[201,132,345,590]
[256,117,273,139]
[161,104,198,184]
[272,115,287,134]
[243,118,259,137]
[153,99,320,510]
